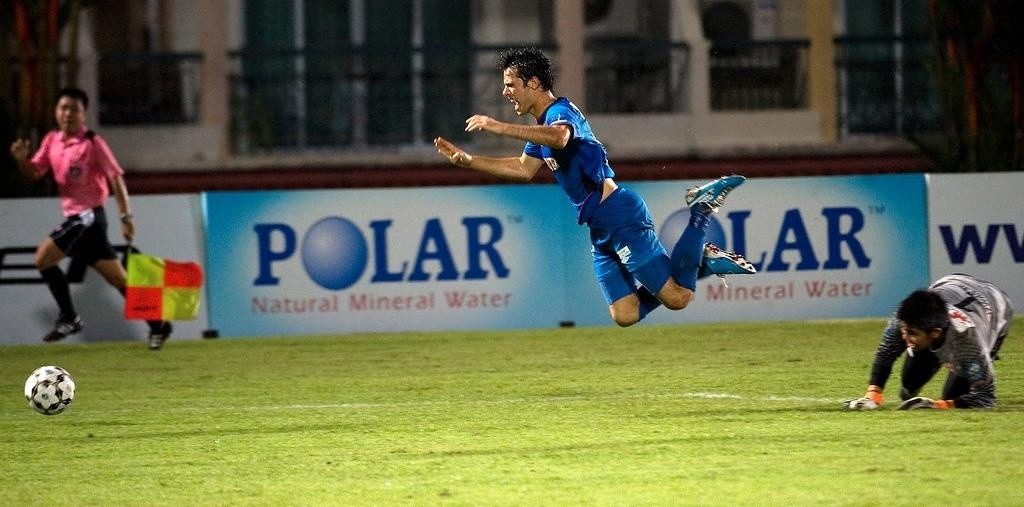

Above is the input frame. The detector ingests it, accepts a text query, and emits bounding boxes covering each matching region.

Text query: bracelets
[120,212,135,223]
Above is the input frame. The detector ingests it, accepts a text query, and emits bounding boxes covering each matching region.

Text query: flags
[123,255,208,322]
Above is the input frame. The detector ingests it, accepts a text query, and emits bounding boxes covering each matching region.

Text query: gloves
[844,398,878,410]
[897,397,950,409]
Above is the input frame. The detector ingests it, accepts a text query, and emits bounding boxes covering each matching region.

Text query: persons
[846,274,1017,413]
[432,43,757,326]
[11,85,173,350]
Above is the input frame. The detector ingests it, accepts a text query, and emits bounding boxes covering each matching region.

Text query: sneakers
[686,175,746,212]
[149,322,171,349]
[43,315,83,340]
[704,242,757,278]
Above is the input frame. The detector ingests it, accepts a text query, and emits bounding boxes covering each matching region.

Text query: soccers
[24,365,75,415]
